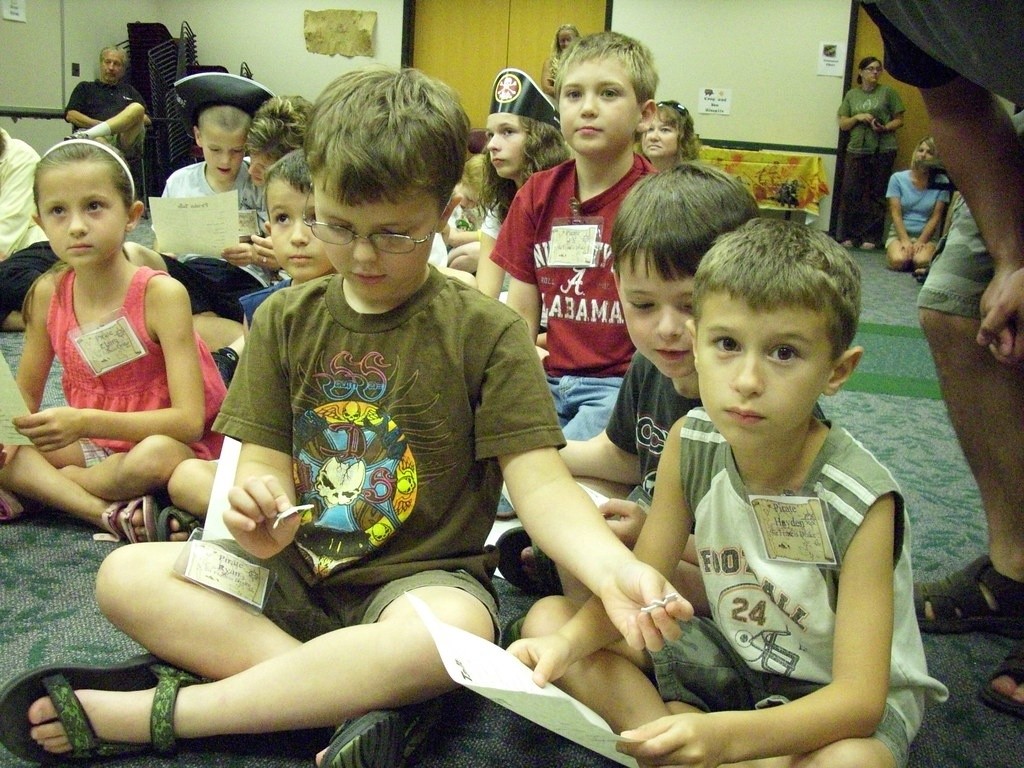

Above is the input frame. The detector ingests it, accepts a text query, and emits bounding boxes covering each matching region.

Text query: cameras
[862,118,885,126]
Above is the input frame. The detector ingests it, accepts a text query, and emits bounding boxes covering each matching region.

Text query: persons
[0,67,694,768]
[0,46,336,551]
[427,0,1023,768]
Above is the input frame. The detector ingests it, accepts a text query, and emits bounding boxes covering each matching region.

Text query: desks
[696,145,831,225]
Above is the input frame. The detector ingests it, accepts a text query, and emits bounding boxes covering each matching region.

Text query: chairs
[70,19,281,221]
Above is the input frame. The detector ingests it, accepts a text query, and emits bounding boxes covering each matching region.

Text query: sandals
[0,654,209,765]
[0,486,36,521]
[916,556,1024,633]
[315,689,466,768]
[93,495,158,545]
[497,527,564,594]
[981,638,1024,718]
[155,506,205,542]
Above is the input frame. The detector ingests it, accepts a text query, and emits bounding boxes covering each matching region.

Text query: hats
[489,68,560,130]
[174,72,276,122]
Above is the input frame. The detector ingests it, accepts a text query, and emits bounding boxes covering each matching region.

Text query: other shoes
[843,241,855,249]
[64,133,89,140]
[210,348,239,386]
[860,243,876,250]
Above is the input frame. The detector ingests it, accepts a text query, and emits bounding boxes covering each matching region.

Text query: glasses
[303,184,453,255]
[656,102,686,118]
[865,67,883,74]
